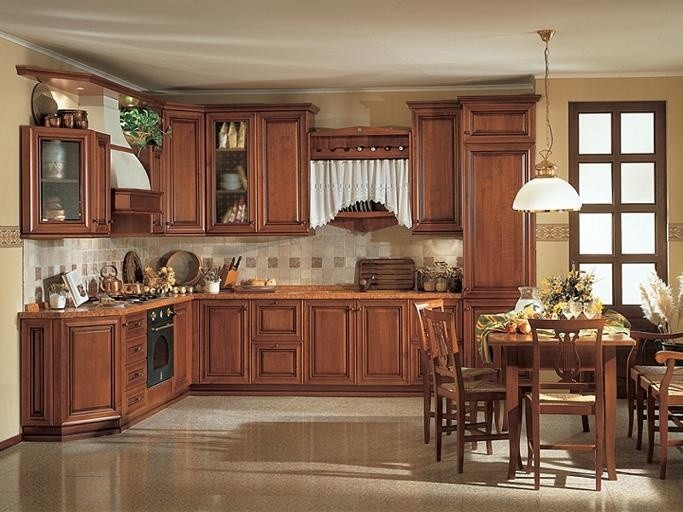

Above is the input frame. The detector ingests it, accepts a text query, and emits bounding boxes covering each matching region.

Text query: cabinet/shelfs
[305,301,405,388]
[206,103,319,236]
[251,300,306,386]
[406,98,461,235]
[16,310,146,442]
[19,124,112,239]
[191,300,252,385]
[172,298,193,404]
[109,102,205,238]
[457,93,542,299]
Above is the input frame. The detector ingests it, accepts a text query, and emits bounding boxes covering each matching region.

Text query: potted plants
[201,265,222,295]
[119,107,173,157]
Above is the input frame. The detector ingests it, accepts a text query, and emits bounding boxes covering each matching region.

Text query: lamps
[508,28,581,214]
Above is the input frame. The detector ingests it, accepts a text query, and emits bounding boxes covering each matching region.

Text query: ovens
[145,320,174,418]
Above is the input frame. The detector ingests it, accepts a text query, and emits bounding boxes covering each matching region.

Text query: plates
[231,286,274,292]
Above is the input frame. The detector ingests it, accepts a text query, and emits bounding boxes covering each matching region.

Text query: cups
[220,174,240,191]
[206,280,218,295]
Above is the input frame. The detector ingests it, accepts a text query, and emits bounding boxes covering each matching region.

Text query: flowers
[537,264,604,320]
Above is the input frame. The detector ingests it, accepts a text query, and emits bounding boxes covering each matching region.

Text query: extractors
[76,94,164,217]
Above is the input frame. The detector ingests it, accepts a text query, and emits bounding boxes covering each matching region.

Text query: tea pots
[515,286,544,313]
[98,265,121,295]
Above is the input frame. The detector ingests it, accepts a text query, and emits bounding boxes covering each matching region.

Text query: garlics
[143,266,194,294]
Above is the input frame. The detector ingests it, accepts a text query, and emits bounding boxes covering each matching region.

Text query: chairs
[523,318,608,490]
[627,331,683,480]
[414,300,522,473]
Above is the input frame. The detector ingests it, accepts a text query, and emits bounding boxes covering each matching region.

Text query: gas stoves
[110,294,174,323]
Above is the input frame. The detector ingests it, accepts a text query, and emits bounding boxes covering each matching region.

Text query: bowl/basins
[42,110,87,129]
[122,283,143,296]
[163,250,202,286]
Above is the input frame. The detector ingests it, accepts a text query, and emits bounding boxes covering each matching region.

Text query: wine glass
[561,302,596,336]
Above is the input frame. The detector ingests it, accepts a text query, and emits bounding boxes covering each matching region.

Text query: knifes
[228,255,240,270]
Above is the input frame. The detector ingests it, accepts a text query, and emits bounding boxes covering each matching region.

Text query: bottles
[49,283,66,309]
[418,260,460,292]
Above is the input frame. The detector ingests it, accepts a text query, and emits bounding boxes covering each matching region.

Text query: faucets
[359,275,375,291]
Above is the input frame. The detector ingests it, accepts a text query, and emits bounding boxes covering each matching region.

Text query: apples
[506,322,517,334]
[519,323,531,334]
